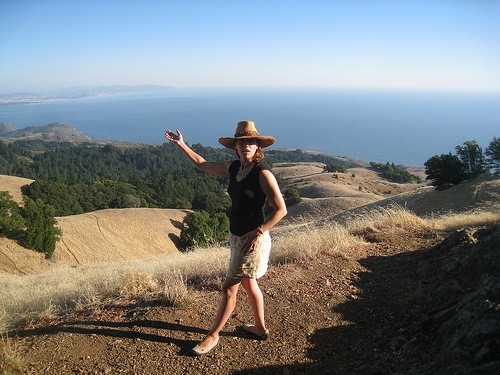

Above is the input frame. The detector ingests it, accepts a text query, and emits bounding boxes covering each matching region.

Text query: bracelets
[258,227,263,234]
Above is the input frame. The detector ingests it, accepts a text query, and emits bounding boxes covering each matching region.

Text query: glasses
[234,139,260,145]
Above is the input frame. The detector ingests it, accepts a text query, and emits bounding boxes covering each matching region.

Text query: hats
[218,121,275,149]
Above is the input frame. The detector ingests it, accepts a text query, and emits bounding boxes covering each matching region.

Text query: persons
[164,121,288,355]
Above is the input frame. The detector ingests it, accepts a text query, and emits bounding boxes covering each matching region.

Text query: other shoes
[241,324,270,339]
[190,333,219,356]
